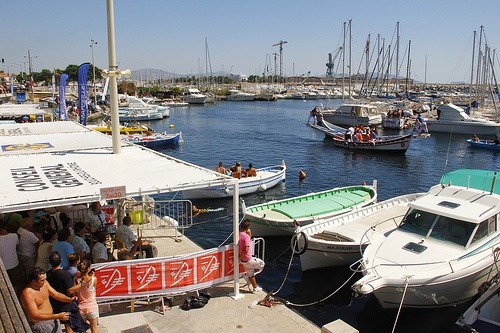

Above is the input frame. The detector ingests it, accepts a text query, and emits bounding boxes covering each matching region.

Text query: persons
[348,124,379,142]
[0,200,115,286]
[147,128,152,135]
[308,107,319,125]
[387,108,405,120]
[21,266,79,333]
[416,114,424,124]
[472,135,479,142]
[215,161,256,179]
[67,259,99,333]
[240,219,263,293]
[44,251,76,333]
[116,216,154,259]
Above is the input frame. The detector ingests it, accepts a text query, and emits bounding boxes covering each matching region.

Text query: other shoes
[253,286,262,292]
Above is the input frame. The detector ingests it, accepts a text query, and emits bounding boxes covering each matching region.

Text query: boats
[455,280,500,333]
[239,179,378,239]
[352,124,500,310]
[295,192,426,274]
[181,160,287,200]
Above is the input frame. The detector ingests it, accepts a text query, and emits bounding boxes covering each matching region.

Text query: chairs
[452,226,465,236]
[114,238,144,258]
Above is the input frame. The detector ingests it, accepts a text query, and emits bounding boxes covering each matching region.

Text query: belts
[241,260,248,262]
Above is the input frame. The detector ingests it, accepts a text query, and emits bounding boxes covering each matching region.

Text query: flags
[59,74,69,121]
[11,77,13,95]
[51,74,55,101]
[77,63,91,127]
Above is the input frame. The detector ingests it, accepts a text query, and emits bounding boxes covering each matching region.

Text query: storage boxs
[130,210,142,224]
[102,208,116,223]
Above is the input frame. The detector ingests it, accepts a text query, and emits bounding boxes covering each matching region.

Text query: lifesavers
[163,215,178,227]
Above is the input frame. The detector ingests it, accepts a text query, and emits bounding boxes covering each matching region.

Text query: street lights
[89,38,97,107]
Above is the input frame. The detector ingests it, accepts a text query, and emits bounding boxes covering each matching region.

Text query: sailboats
[0,0,500,156]
